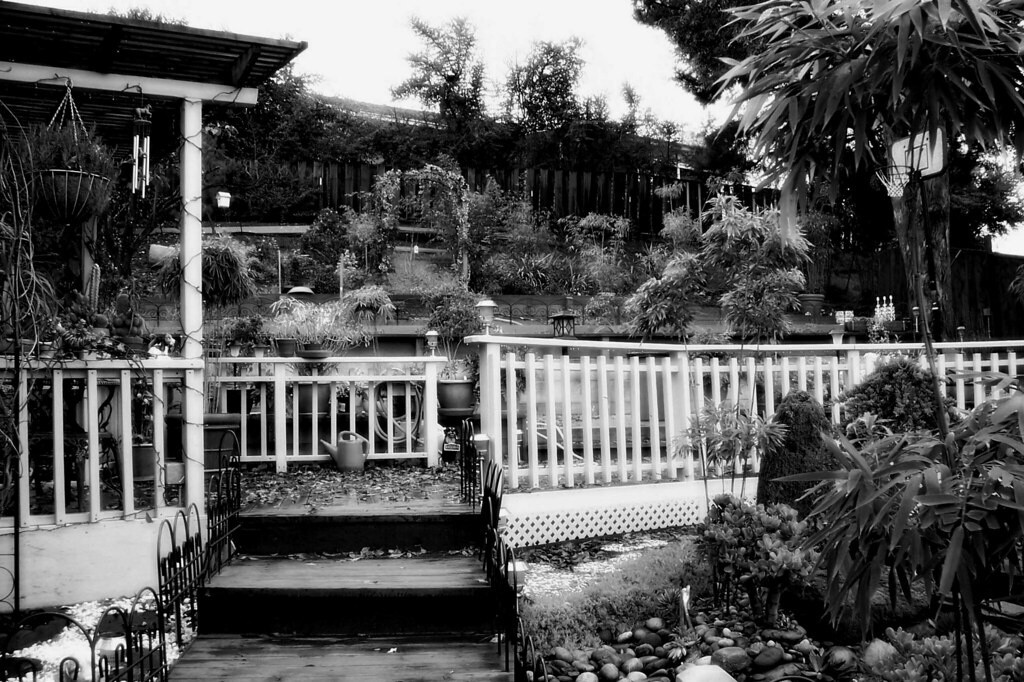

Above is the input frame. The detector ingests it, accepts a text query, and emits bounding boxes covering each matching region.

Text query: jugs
[320,431,370,472]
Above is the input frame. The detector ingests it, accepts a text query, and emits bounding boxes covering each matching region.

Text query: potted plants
[625,276,676,422]
[18,122,137,230]
[404,256,489,408]
[464,350,528,456]
[132,283,397,493]
[796,208,840,314]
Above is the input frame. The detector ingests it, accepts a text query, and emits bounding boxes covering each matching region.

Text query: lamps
[475,300,498,333]
[828,329,846,345]
[216,191,231,207]
[250,339,270,359]
[983,308,991,316]
[550,310,581,340]
[425,331,439,355]
[470,434,489,457]
[227,339,243,356]
[957,326,966,336]
[912,306,921,318]
